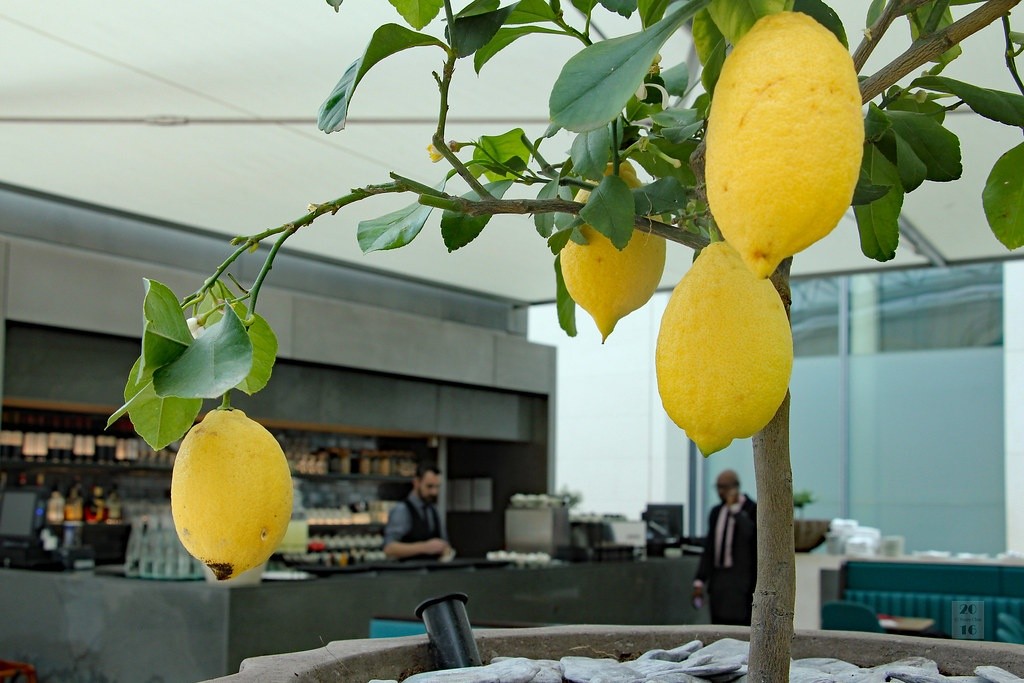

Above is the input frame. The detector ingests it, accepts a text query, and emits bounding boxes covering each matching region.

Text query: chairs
[822,600,880,633]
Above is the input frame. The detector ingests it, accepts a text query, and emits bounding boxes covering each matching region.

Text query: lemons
[171,404,292,580]
[559,160,666,345]
[654,242,793,458]
[703,9,866,280]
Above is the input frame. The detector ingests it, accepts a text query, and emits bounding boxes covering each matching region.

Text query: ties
[719,510,730,566]
[423,505,430,530]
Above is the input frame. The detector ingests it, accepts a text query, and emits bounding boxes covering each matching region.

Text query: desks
[879,616,935,633]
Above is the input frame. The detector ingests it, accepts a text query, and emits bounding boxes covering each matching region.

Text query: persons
[383,463,452,563]
[692,470,757,627]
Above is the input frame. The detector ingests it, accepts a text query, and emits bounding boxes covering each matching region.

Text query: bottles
[0,410,418,577]
